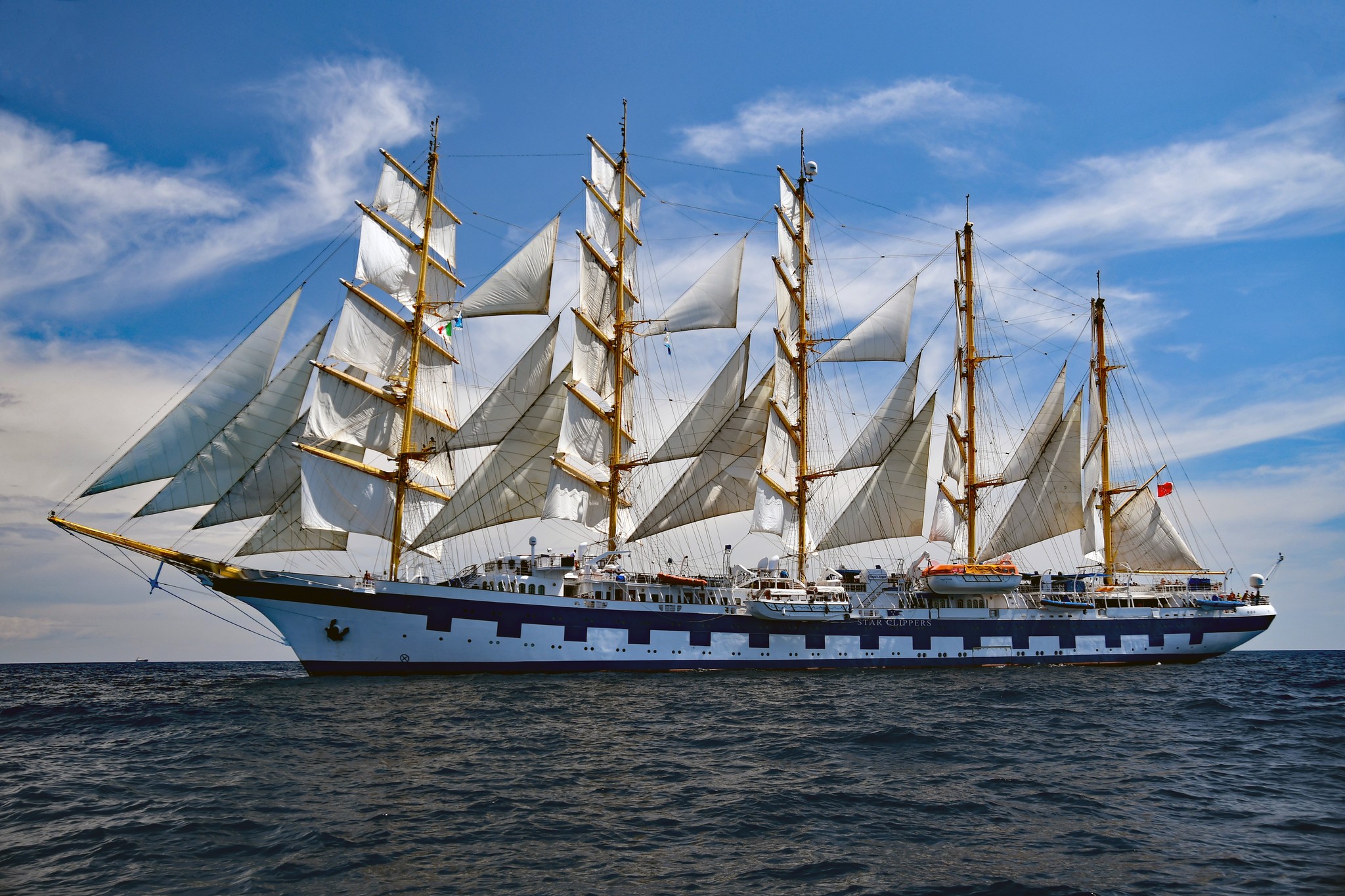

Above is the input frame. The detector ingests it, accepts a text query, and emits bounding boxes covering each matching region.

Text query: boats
[915,559,1023,595]
[742,585,854,620]
[1039,599,1095,614]
[1195,599,1246,611]
[656,572,707,587]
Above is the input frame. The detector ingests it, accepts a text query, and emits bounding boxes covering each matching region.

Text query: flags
[664,331,671,357]
[1157,482,1174,497]
[438,311,462,336]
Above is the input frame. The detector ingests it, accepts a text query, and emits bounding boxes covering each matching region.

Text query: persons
[363,571,370,586]
[1175,579,1179,584]
[920,601,926,608]
[570,550,576,557]
[1167,579,1171,584]
[1115,579,1118,585]
[1243,590,1251,600]
[575,562,579,566]
[1255,591,1263,605]
[472,565,476,574]
[1160,578,1166,584]
[1237,593,1241,599]
[1230,593,1235,601]
[1096,604,1100,608]
[893,583,895,587]
[808,596,815,603]
[1250,592,1255,601]
[1137,602,1142,607]
[513,567,520,575]
[1133,582,1135,585]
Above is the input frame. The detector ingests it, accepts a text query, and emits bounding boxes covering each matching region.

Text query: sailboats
[47,99,1285,668]
